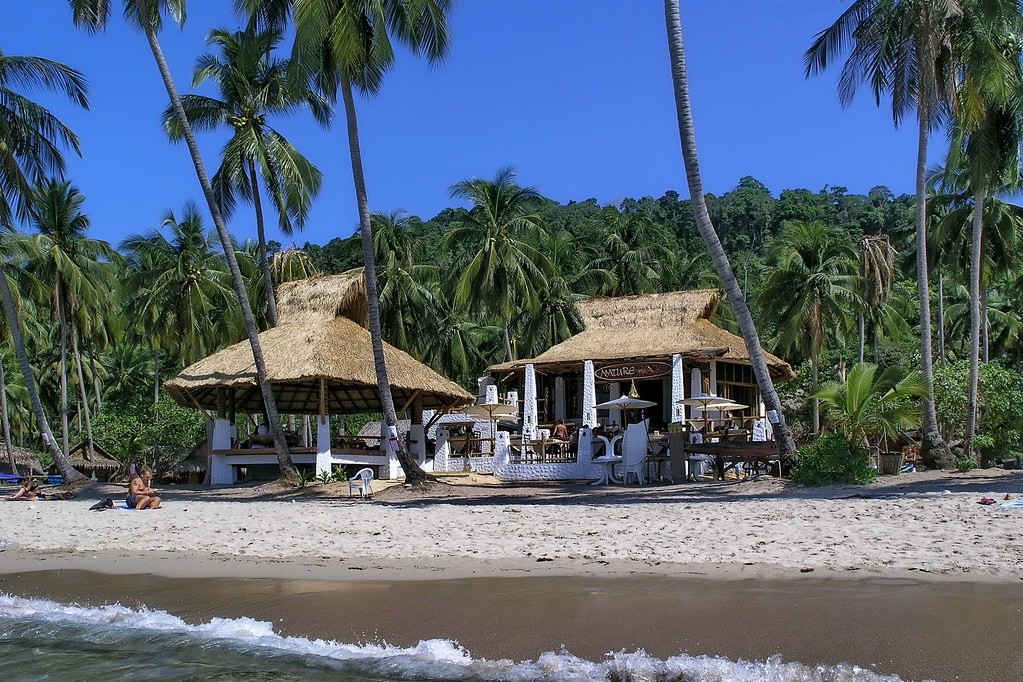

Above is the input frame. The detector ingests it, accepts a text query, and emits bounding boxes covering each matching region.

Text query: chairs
[523,420,760,487]
[349,468,374,499]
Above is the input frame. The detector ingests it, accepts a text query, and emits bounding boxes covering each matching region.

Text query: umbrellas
[695,402,749,426]
[592,395,657,430]
[462,400,521,452]
[676,393,736,428]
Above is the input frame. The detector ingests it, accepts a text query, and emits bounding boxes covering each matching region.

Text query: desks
[591,438,607,456]
[531,439,563,462]
[597,435,622,460]
[591,460,625,485]
[449,435,470,457]
[648,434,670,458]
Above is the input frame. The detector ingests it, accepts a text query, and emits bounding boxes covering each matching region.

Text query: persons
[551,418,605,444]
[126,464,161,510]
[258,420,269,435]
[10,478,41,500]
